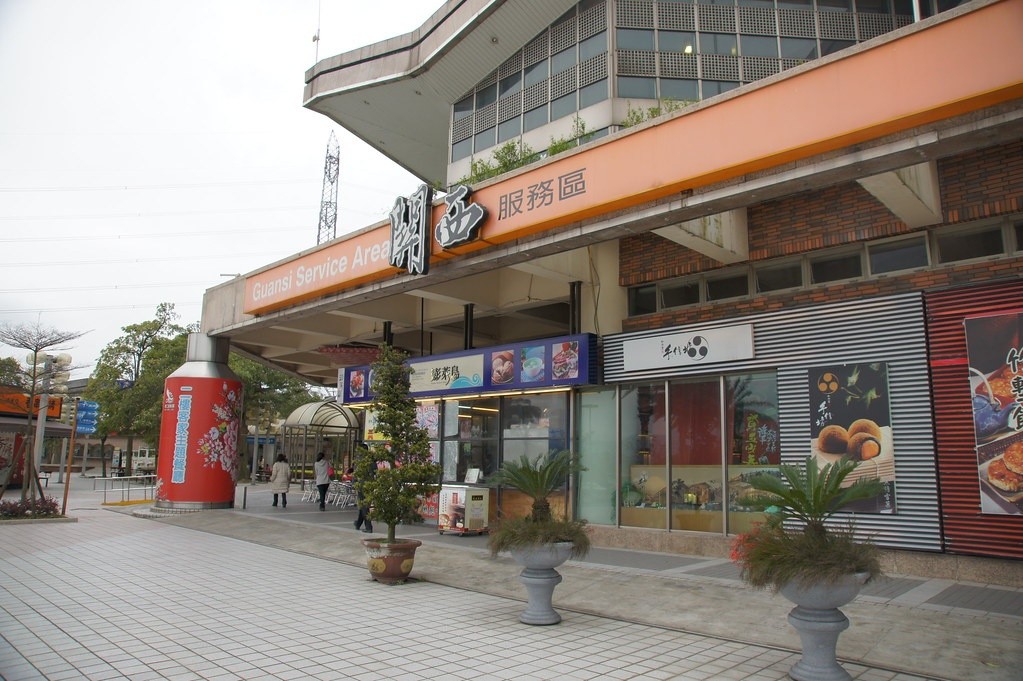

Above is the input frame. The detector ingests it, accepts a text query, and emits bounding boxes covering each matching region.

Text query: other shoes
[362,529,372,533]
[320,508,325,511]
[354,520,359,530]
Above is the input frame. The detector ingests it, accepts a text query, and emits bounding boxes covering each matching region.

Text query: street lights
[25,353,73,500]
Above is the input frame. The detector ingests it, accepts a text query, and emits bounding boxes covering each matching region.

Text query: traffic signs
[76,401,100,433]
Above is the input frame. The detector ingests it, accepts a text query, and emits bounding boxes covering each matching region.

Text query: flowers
[729,513,783,589]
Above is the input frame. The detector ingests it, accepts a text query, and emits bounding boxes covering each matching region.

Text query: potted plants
[737,454,885,681]
[354,340,442,585]
[487,505,595,624]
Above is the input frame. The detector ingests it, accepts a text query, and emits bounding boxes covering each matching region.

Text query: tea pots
[969,367,1020,439]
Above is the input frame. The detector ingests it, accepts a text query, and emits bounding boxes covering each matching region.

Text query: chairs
[302,480,357,509]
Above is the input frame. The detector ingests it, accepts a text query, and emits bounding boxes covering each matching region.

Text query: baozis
[492,358,514,382]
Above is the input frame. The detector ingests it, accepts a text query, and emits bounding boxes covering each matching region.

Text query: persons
[353,444,377,532]
[314,452,331,511]
[270,454,291,507]
[257,455,265,471]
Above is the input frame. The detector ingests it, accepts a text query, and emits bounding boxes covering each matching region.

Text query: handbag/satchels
[328,462,335,481]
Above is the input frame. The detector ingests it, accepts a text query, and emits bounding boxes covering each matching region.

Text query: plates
[350,372,359,396]
[979,453,1023,503]
[811,426,895,488]
[491,374,513,384]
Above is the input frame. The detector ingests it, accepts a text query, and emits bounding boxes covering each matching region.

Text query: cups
[522,357,545,378]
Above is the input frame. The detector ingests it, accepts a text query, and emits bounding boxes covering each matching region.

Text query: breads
[988,441,1023,493]
[983,361,1023,398]
[817,419,881,461]
